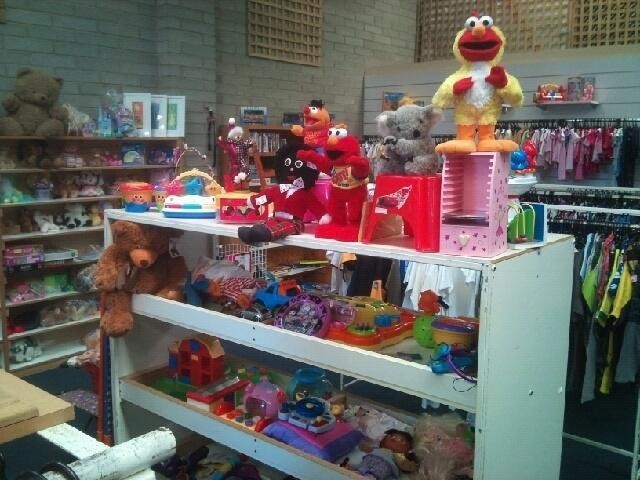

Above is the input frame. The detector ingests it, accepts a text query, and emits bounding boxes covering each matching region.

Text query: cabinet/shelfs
[0,135,186,378]
[219,125,292,192]
[104,208,575,480]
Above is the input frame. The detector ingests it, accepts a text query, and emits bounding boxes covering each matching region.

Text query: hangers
[434,133,454,145]
[494,118,639,129]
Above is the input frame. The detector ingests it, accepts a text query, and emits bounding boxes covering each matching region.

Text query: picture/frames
[123,93,185,137]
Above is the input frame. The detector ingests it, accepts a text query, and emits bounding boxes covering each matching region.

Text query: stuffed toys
[0,9,525,339]
[218,14,524,245]
[8,337,42,365]
[2,66,70,137]
[342,428,418,480]
[96,220,185,337]
[2,140,104,236]
